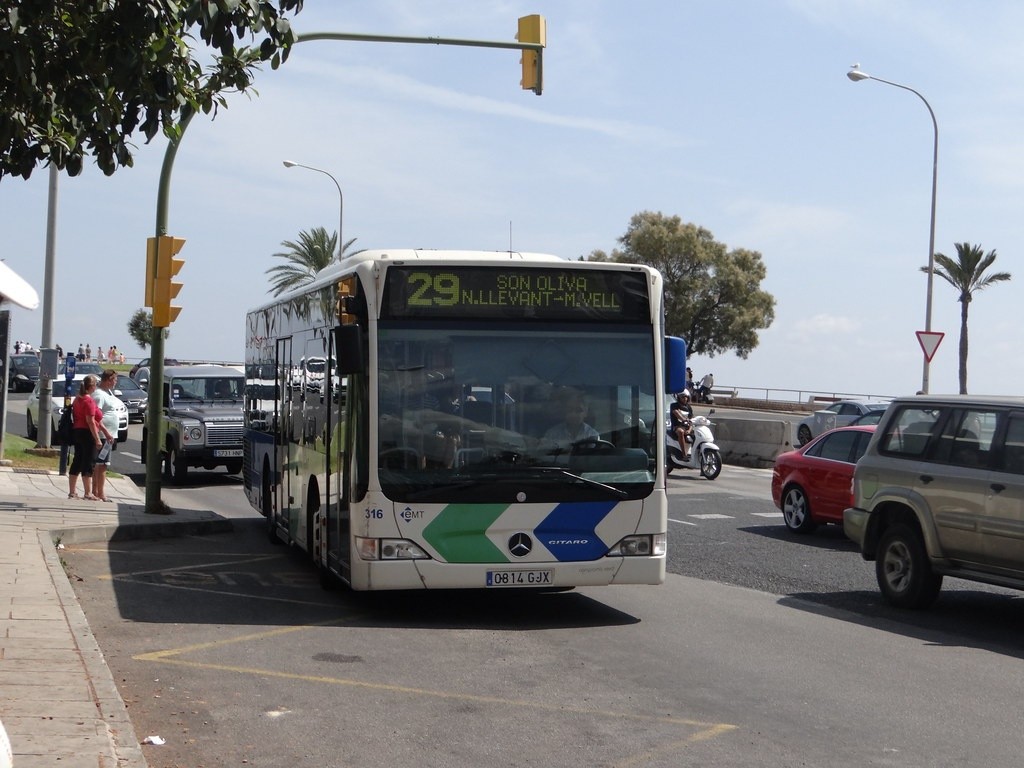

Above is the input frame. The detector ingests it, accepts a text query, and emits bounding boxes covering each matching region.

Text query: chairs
[208,381,222,399]
[171,384,184,398]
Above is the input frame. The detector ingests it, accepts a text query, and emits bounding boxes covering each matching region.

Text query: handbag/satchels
[59,405,75,442]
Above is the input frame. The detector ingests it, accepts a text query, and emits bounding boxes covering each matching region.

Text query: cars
[795,395,942,458]
[134,366,150,394]
[771,423,907,536]
[129,357,181,379]
[245,355,348,437]
[99,374,149,423]
[8,349,106,393]
[465,387,519,405]
[27,373,130,445]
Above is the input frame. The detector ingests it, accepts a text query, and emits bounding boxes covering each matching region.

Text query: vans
[844,393,1024,611]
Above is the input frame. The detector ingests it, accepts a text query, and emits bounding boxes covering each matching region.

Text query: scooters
[692,381,715,404]
[646,408,722,481]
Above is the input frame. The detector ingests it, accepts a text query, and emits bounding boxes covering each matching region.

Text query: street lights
[847,70,938,395]
[282,160,342,261]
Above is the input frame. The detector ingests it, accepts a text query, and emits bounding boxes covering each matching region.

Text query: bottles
[98,439,112,460]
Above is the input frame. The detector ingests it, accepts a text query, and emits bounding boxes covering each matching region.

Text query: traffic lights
[153,236,187,327]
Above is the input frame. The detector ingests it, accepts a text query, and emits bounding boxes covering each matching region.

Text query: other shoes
[103,498,112,502]
[68,493,83,499]
[83,493,103,502]
[682,457,689,461]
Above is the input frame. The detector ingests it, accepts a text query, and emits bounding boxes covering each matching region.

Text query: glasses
[566,407,586,414]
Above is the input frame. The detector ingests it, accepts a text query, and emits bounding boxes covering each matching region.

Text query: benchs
[903,422,982,463]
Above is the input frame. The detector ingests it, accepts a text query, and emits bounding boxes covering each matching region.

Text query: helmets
[678,388,689,397]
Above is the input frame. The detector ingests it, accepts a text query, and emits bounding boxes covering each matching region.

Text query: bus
[242,248,687,592]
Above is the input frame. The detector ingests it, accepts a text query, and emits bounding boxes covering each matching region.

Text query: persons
[25,342,31,350]
[78,343,85,362]
[108,345,118,364]
[670,388,693,462]
[96,346,105,364]
[696,373,713,393]
[91,369,119,502]
[686,367,692,387]
[68,376,115,502]
[120,352,124,363]
[19,341,25,354]
[14,341,19,354]
[544,391,599,448]
[56,343,63,357]
[85,344,91,362]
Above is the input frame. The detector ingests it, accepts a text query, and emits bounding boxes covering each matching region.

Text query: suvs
[141,365,244,486]
